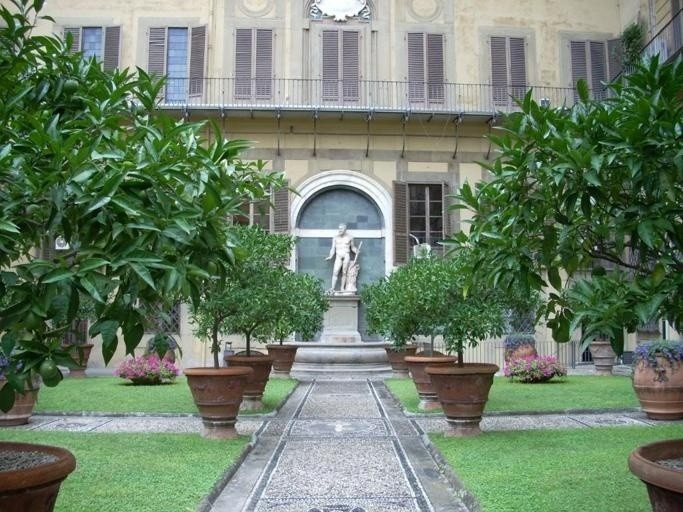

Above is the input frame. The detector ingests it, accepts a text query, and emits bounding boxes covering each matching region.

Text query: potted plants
[0,0,331,511]
[358,62,682,512]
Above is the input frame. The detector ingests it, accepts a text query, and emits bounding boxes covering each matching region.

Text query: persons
[324,223,359,292]
